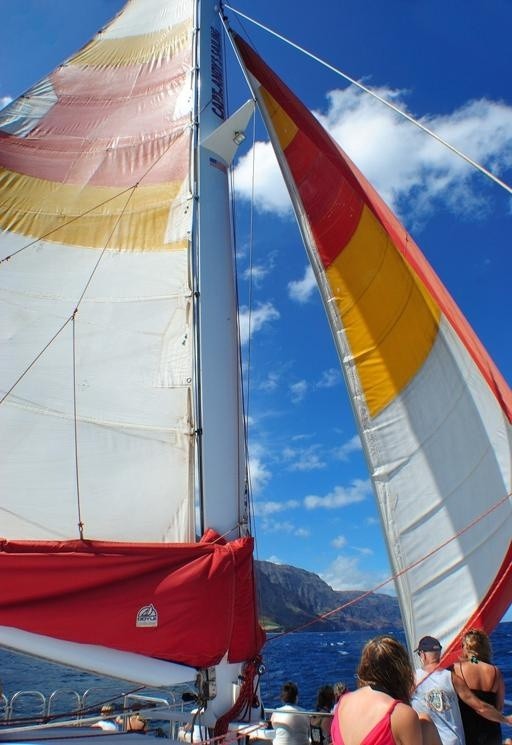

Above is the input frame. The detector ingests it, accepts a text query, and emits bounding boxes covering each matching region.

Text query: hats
[413,636,441,653]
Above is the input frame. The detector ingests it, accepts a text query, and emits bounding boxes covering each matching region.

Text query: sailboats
[0,0,511,745]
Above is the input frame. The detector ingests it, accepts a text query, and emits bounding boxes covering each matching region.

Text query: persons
[90,704,121,733]
[404,635,511,745]
[329,680,347,713]
[116,703,149,735]
[329,634,444,745]
[269,679,314,745]
[308,684,338,745]
[176,692,211,745]
[445,628,507,745]
